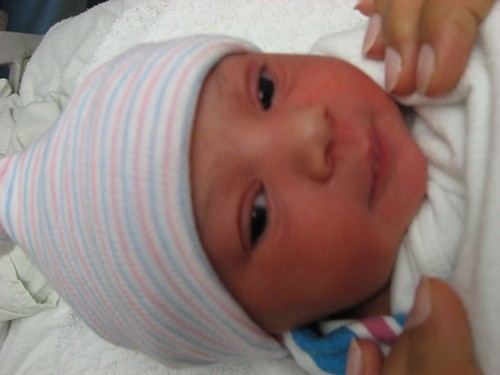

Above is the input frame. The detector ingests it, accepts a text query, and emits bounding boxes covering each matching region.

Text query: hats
[0,34,288,365]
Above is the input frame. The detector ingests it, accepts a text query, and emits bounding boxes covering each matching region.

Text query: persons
[346,0,495,375]
[1,0,499,374]
